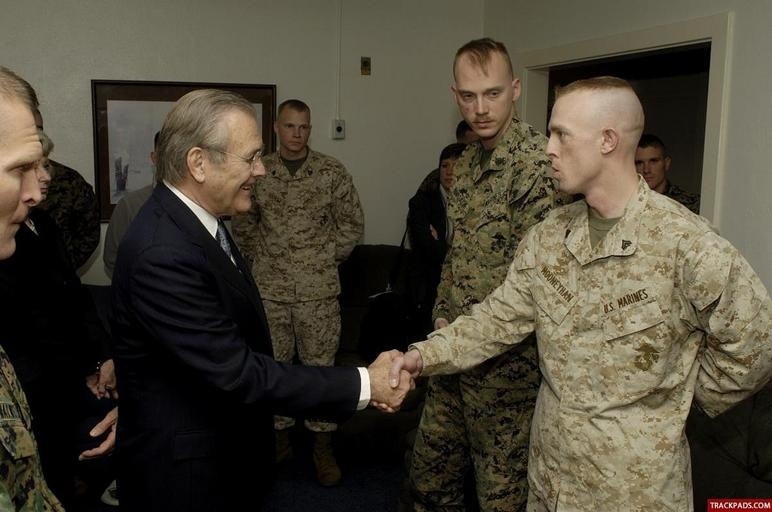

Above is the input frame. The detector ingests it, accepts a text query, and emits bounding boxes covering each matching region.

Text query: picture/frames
[91,79,278,222]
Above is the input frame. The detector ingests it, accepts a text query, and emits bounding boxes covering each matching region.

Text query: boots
[274,429,294,465]
[312,432,342,487]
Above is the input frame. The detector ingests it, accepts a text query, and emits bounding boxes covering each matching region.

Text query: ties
[216,223,232,259]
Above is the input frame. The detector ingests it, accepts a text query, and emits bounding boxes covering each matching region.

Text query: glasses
[196,143,266,165]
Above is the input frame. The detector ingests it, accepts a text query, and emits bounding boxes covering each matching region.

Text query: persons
[369,77,771,512]
[416,119,485,192]
[30,108,101,272]
[404,37,573,512]
[403,144,471,347]
[79,87,417,509]
[630,133,700,217]
[0,126,120,512]
[225,98,367,487]
[103,132,162,282]
[0,67,72,512]
